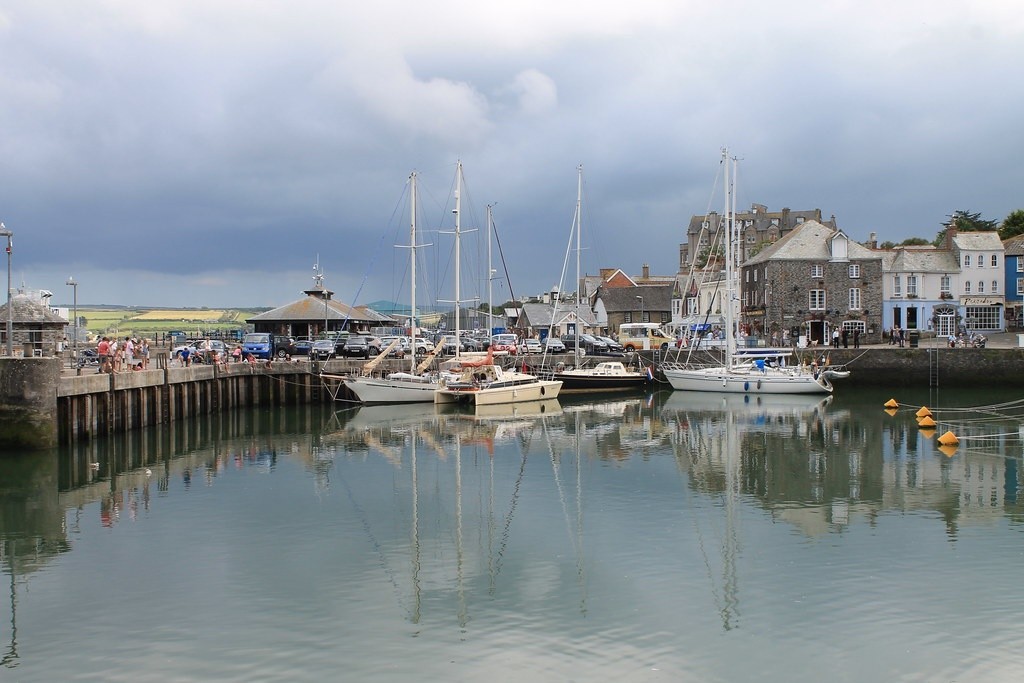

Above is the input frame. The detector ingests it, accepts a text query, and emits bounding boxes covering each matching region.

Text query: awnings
[690,324,710,330]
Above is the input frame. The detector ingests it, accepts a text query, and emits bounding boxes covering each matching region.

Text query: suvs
[365,336,384,356]
[379,335,403,357]
[561,333,609,353]
[436,334,466,356]
[342,335,370,360]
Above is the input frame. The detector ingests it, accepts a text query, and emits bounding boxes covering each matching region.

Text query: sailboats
[318,145,853,406]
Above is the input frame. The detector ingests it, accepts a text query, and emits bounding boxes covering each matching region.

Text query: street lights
[65,275,79,362]
[636,295,643,322]
[0,221,14,357]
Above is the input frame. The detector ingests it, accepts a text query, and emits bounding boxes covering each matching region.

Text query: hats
[205,336,210,339]
[131,338,138,343]
[835,328,839,331]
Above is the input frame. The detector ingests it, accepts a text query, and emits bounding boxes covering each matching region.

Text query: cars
[522,338,543,354]
[408,336,435,355]
[309,339,337,360]
[460,336,485,352]
[602,336,625,353]
[172,339,233,360]
[542,337,566,354]
[295,339,314,357]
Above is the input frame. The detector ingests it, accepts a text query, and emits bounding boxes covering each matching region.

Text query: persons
[833,327,860,348]
[97,336,150,374]
[734,327,789,348]
[180,348,191,367]
[194,337,273,374]
[511,331,561,356]
[460,332,474,339]
[707,328,723,340]
[889,325,905,348]
[949,326,988,348]
[607,330,617,341]
[285,354,300,364]
[63,337,68,349]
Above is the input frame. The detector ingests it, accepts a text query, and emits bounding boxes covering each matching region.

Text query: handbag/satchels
[135,366,141,370]
[137,362,143,368]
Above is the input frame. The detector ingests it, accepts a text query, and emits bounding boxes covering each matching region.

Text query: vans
[488,333,519,346]
[272,336,297,357]
[240,332,277,361]
[619,322,679,352]
